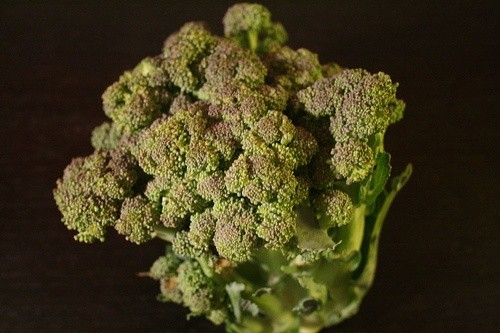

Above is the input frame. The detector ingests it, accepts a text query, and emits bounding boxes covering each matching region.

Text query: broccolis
[51,3,413,333]
